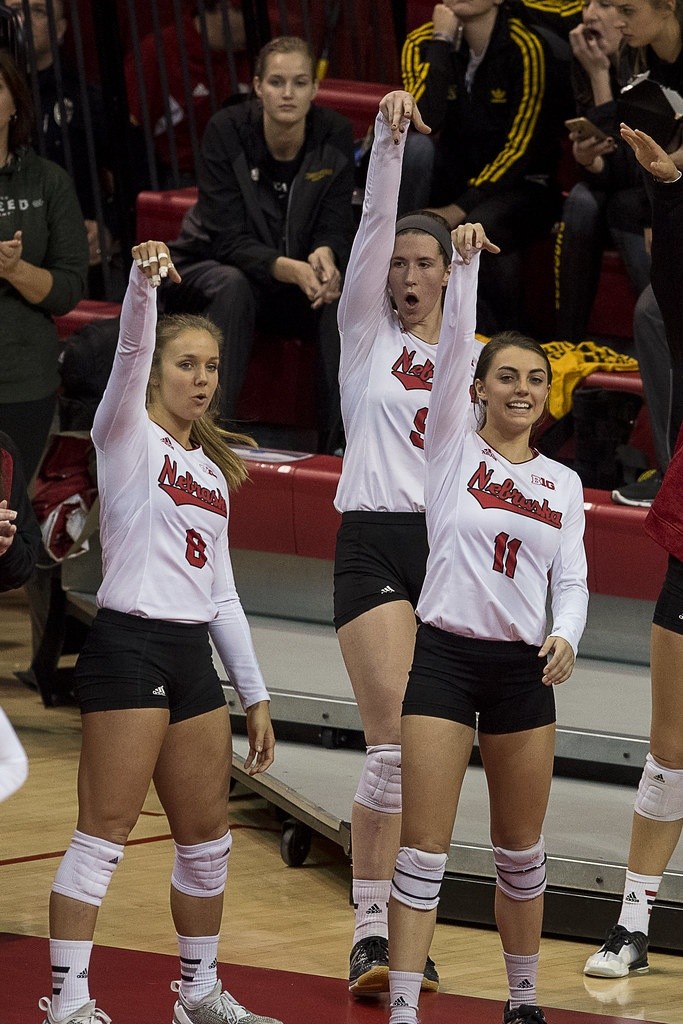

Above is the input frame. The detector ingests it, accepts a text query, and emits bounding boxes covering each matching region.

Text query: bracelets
[433,33,454,43]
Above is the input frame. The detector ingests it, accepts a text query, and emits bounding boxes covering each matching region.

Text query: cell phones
[565,115,617,147]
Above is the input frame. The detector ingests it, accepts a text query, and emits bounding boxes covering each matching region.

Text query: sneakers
[584,924,652,977]
[348,936,390,994]
[502,999,547,1024]
[422,955,441,991]
[611,469,665,508]
[169,978,283,1024]
[38,996,112,1024]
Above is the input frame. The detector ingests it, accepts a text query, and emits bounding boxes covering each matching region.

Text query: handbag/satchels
[35,495,103,594]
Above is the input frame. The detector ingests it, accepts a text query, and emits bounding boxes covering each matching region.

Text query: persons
[584,121,683,978]
[0,0,245,487]
[39,240,281,1024]
[356,0,683,507]
[389,223,589,1024]
[0,431,44,802]
[157,35,355,455]
[337,88,487,991]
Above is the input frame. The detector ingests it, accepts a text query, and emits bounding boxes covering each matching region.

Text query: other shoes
[315,431,345,456]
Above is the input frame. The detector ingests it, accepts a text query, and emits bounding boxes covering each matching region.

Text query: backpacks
[56,317,120,428]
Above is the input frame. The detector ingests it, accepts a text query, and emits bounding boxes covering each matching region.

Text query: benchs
[0,0,655,664]
